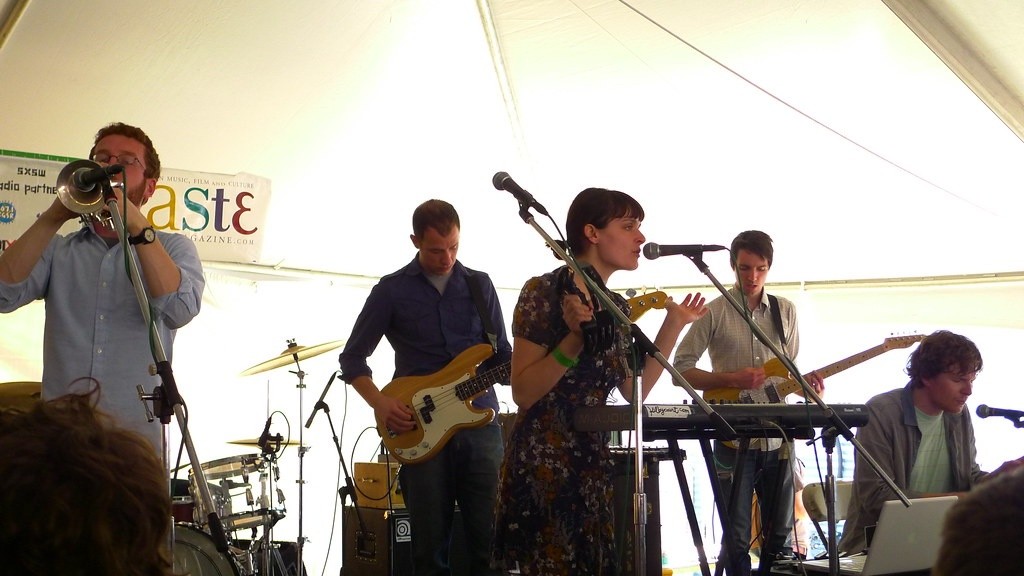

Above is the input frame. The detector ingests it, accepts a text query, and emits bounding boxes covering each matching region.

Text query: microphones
[71,163,124,192]
[492,171,548,216]
[642,242,725,260]
[257,416,272,446]
[976,404,1024,418]
[304,371,337,428]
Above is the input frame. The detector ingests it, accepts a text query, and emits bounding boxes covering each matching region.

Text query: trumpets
[56,159,111,216]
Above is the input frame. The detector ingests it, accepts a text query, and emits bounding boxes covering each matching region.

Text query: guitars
[373,284,669,464]
[700,331,927,448]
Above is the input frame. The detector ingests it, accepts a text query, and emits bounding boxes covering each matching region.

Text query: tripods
[244,472,289,576]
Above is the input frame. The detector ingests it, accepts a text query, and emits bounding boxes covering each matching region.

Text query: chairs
[802,480,854,552]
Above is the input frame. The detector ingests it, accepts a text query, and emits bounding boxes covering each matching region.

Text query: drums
[167,522,253,576]
[167,479,226,530]
[186,454,286,532]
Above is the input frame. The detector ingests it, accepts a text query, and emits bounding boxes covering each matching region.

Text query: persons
[491,188,709,576]
[931,464,1024,576]
[0,123,206,462]
[0,390,173,576]
[672,230,824,576]
[837,330,1024,555]
[337,199,512,576]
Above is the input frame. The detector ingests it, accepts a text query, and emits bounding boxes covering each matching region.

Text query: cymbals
[241,339,346,377]
[226,435,303,449]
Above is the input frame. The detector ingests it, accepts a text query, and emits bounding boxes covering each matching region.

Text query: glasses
[93,153,146,173]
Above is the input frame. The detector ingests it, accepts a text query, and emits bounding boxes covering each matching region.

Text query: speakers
[343,506,413,576]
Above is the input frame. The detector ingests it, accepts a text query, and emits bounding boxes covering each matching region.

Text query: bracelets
[553,346,579,368]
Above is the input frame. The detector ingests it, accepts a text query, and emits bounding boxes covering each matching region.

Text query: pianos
[573,402,871,576]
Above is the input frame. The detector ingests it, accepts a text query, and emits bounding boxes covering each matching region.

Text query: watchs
[128,226,157,246]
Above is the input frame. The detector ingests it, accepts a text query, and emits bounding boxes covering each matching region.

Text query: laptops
[799,495,958,576]
